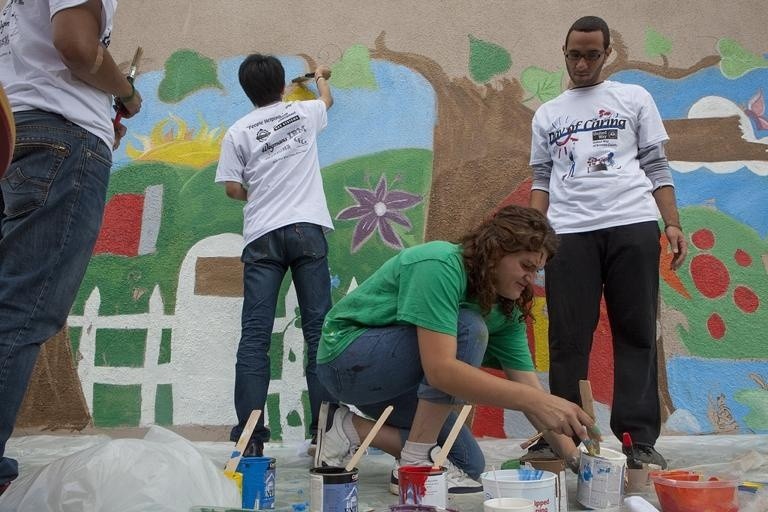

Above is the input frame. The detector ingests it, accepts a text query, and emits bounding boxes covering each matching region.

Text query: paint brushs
[520,429,552,449]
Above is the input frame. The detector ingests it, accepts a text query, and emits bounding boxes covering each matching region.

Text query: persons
[0,0,143,494]
[212,52,336,458]
[314,205,604,501]
[502,17,689,471]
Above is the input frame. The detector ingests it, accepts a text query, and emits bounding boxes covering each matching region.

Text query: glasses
[563,52,606,60]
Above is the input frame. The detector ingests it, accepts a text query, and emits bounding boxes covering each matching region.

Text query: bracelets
[665,223,682,231]
[119,80,135,102]
[316,75,325,83]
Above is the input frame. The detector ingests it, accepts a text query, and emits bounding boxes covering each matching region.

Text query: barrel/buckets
[397,465,448,510]
[308,465,359,512]
[480,467,559,512]
[235,455,275,509]
[577,447,626,511]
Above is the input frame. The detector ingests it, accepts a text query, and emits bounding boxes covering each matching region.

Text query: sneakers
[243,441,263,458]
[390,446,483,500]
[633,446,667,470]
[311,401,358,468]
[519,437,556,460]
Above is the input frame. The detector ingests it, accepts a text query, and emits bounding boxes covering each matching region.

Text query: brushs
[623,433,643,469]
[292,72,315,83]
[114,46,144,129]
